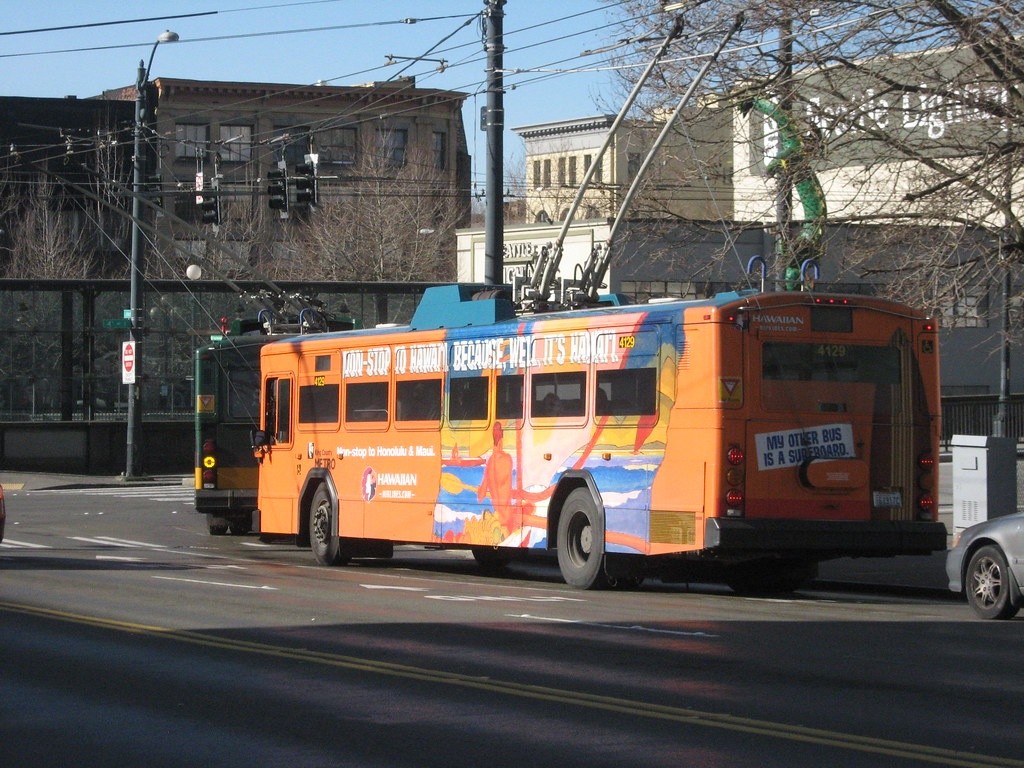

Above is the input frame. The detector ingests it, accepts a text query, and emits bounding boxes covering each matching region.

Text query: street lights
[17,317,36,420]
[124,29,180,480]
[147,295,183,414]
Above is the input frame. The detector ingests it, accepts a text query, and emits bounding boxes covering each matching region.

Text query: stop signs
[120,339,135,386]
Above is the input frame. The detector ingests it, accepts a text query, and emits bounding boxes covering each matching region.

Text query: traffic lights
[295,152,320,205]
[221,314,229,334]
[201,177,222,226]
[267,160,290,219]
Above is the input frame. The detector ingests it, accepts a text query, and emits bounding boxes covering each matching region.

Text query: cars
[0,485,8,544]
[945,512,1024,620]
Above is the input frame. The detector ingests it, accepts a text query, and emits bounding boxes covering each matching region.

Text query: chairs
[596,388,608,416]
[544,393,563,415]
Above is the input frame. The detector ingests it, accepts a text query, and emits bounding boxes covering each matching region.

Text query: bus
[249,280,948,591]
[194,288,378,535]
